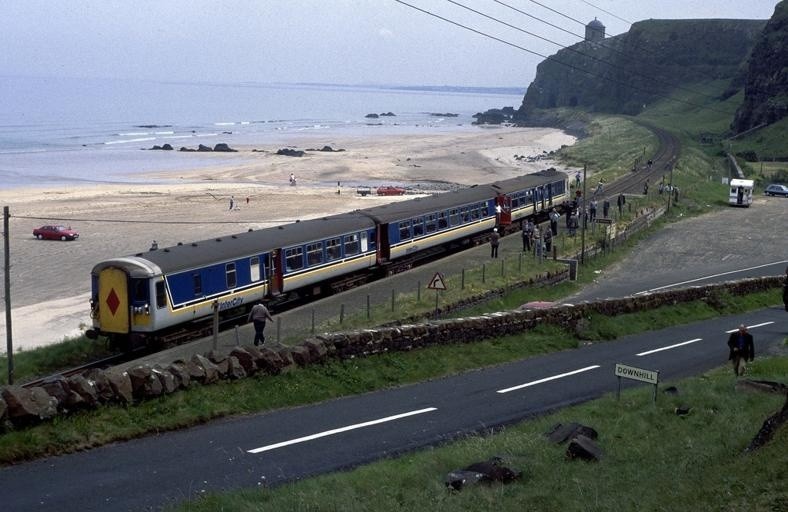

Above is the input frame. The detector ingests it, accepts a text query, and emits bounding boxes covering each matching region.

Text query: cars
[33,224,80,242]
[764,183,788,198]
[376,184,407,197]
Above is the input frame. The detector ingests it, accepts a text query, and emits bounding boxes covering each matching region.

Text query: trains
[85,167,570,359]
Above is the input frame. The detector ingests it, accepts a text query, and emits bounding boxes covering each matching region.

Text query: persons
[494,202,505,225]
[565,192,598,236]
[644,179,650,195]
[491,228,500,258]
[576,171,581,184]
[229,195,234,209]
[647,160,652,169]
[659,182,678,200]
[595,178,604,195]
[727,323,754,378]
[618,195,625,211]
[248,298,274,347]
[603,197,610,218]
[290,173,295,182]
[521,208,559,257]
